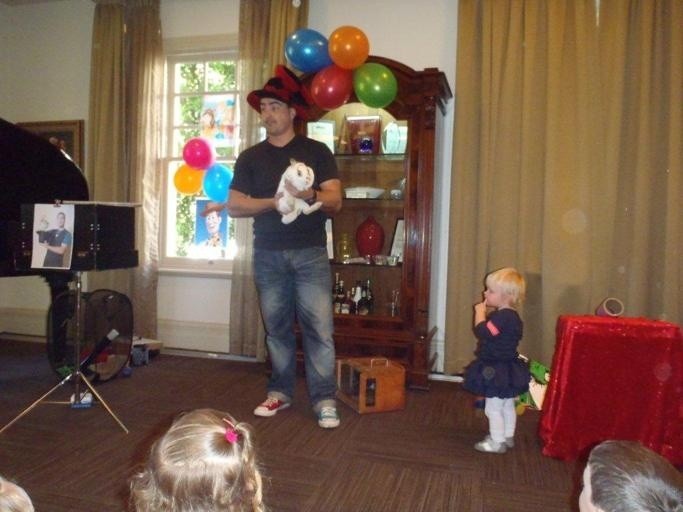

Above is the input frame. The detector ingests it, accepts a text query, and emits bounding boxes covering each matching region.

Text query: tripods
[0,200,136,434]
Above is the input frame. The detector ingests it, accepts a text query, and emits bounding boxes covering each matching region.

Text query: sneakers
[253,397,289,417]
[485,434,514,448]
[318,403,340,429]
[474,440,507,454]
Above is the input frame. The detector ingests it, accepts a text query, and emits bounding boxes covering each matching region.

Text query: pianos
[1,118,139,364]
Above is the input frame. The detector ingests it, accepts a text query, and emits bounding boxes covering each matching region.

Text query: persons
[198,200,226,251]
[36,212,73,268]
[124,407,273,512]
[578,440,683,512]
[461,266,532,455]
[226,63,344,429]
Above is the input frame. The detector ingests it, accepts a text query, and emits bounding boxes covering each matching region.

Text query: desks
[541,315,683,467]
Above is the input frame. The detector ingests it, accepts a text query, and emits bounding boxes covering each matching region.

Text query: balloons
[200,162,235,205]
[182,137,214,171]
[283,25,331,76]
[309,61,354,113]
[327,24,370,71]
[172,163,207,196]
[353,61,399,109]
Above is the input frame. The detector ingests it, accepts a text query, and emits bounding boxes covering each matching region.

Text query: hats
[200,202,225,218]
[246,64,313,122]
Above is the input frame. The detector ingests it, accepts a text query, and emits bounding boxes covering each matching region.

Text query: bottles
[341,290,353,315]
[334,272,341,295]
[339,280,345,302]
[352,287,355,296]
[353,280,361,314]
[366,279,375,313]
[357,285,370,315]
[334,297,341,314]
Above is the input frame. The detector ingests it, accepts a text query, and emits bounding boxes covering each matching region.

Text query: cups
[385,256,399,266]
[372,255,386,264]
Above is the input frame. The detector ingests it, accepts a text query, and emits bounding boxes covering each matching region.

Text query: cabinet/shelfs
[264,54,453,391]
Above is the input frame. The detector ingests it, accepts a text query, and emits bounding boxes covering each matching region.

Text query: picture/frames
[389,217,405,265]
[14,120,84,171]
[307,119,337,155]
[325,217,335,263]
